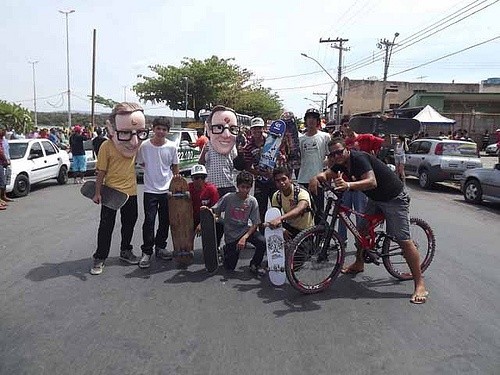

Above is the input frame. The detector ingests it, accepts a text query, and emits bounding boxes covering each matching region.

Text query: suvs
[403,137,483,189]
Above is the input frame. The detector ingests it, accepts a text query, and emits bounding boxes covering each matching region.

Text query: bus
[200,113,252,129]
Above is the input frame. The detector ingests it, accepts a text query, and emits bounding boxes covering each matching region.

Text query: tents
[412,105,456,139]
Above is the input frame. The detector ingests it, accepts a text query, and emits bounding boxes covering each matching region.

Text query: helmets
[305,108,321,116]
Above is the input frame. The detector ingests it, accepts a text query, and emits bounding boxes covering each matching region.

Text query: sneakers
[244,242,256,249]
[155,247,173,260]
[139,251,151,268]
[89,259,105,275]
[119,250,140,264]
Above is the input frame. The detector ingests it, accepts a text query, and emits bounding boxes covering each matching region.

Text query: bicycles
[284,181,436,294]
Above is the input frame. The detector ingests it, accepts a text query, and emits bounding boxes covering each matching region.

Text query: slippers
[410,290,430,304]
[341,264,364,274]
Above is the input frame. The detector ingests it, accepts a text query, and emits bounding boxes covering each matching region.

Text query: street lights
[28,61,39,125]
[59,10,76,127]
[301,53,342,130]
[182,77,188,120]
[381,33,399,114]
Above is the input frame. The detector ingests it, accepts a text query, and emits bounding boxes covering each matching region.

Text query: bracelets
[347,182,350,191]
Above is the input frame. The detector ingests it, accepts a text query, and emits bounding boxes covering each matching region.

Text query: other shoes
[365,256,373,263]
[249,260,266,276]
[0,198,12,209]
[74,179,86,184]
[217,245,226,263]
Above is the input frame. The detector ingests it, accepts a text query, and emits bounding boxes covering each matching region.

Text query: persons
[308,137,429,305]
[496,129,500,144]
[0,102,477,276]
[481,130,490,151]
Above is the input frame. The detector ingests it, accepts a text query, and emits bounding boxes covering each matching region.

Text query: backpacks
[277,182,320,220]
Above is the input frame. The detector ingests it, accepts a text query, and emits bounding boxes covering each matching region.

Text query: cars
[61,139,96,175]
[486,143,500,155]
[460,164,500,205]
[136,131,201,178]
[5,138,70,196]
[378,134,398,158]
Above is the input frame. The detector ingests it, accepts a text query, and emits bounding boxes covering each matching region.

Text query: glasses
[192,176,206,180]
[329,147,345,157]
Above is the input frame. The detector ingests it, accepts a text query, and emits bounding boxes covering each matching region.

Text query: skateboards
[200,206,221,273]
[167,177,195,264]
[257,120,287,184]
[261,207,286,285]
[81,180,129,210]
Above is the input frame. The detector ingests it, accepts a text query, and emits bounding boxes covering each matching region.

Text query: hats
[191,165,208,175]
[280,111,295,120]
[74,125,82,132]
[250,117,264,127]
